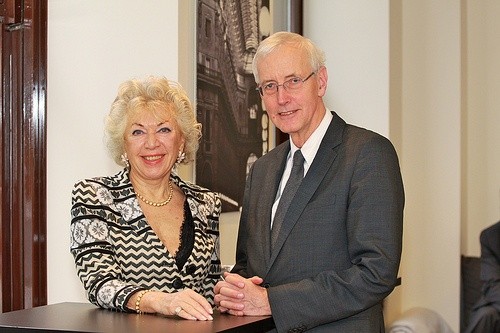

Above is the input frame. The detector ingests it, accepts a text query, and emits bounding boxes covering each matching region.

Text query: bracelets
[135,289,155,314]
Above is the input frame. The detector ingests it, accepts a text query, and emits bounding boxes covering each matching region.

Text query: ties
[269,150,305,252]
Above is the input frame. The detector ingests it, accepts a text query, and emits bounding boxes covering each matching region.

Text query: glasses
[256,71,316,97]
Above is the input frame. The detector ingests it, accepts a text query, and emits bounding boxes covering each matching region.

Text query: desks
[0,302,277,333]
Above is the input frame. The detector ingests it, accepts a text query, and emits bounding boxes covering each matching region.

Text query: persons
[460,221,500,333]
[216,31,405,332]
[70,75,232,323]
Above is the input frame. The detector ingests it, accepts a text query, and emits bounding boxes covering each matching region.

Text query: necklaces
[133,181,174,207]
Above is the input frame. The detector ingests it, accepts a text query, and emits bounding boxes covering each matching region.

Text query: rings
[174,307,185,315]
